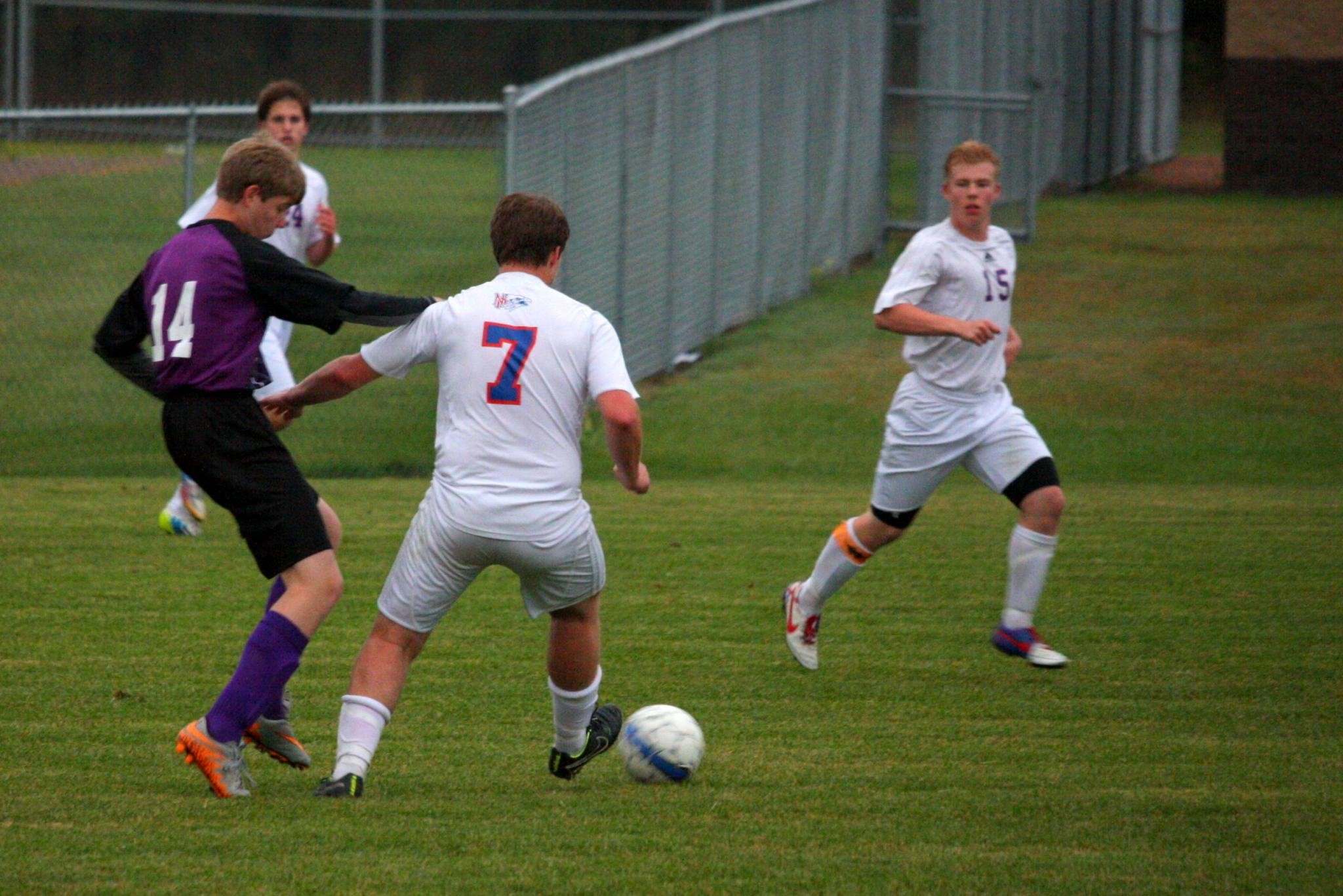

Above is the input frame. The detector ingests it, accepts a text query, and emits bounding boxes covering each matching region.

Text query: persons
[153,79,342,538]
[782,139,1074,671]
[87,130,442,802]
[255,192,653,798]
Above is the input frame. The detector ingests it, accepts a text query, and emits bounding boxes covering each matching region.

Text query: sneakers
[181,478,208,523]
[159,508,203,536]
[992,625,1069,668]
[243,687,317,770]
[175,716,258,797]
[783,580,822,670]
[313,772,363,798]
[548,702,623,780]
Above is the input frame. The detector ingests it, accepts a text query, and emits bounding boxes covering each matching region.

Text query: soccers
[618,702,708,787]
